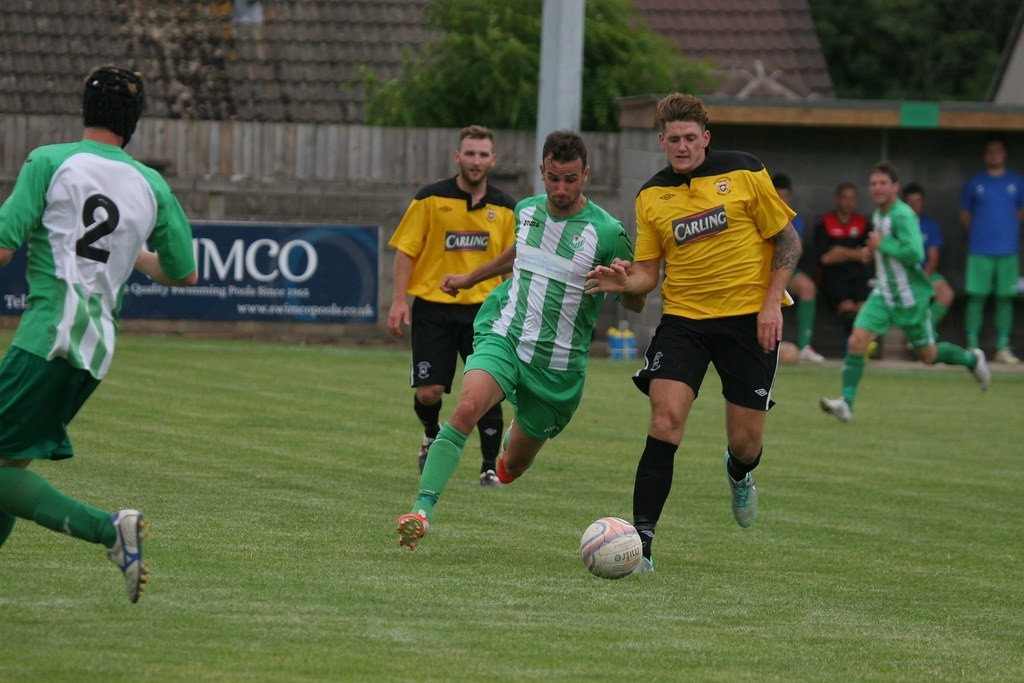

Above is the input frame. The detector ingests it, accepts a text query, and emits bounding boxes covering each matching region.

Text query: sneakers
[725,451,757,528]
[107,509,148,604]
[631,556,654,574]
[969,348,991,389]
[821,396,852,421]
[496,418,517,485]
[419,422,441,475]
[480,469,500,485]
[397,514,428,552]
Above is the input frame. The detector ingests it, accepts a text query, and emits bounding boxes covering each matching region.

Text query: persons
[768,175,954,363]
[819,162,990,424]
[582,92,801,574]
[1,63,197,603]
[386,125,517,489]
[956,138,1024,363]
[397,130,646,552]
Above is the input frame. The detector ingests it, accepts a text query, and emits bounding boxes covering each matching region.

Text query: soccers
[580,517,643,580]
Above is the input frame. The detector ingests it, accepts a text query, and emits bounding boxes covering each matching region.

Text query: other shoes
[798,345,823,362]
[995,351,1019,363]
